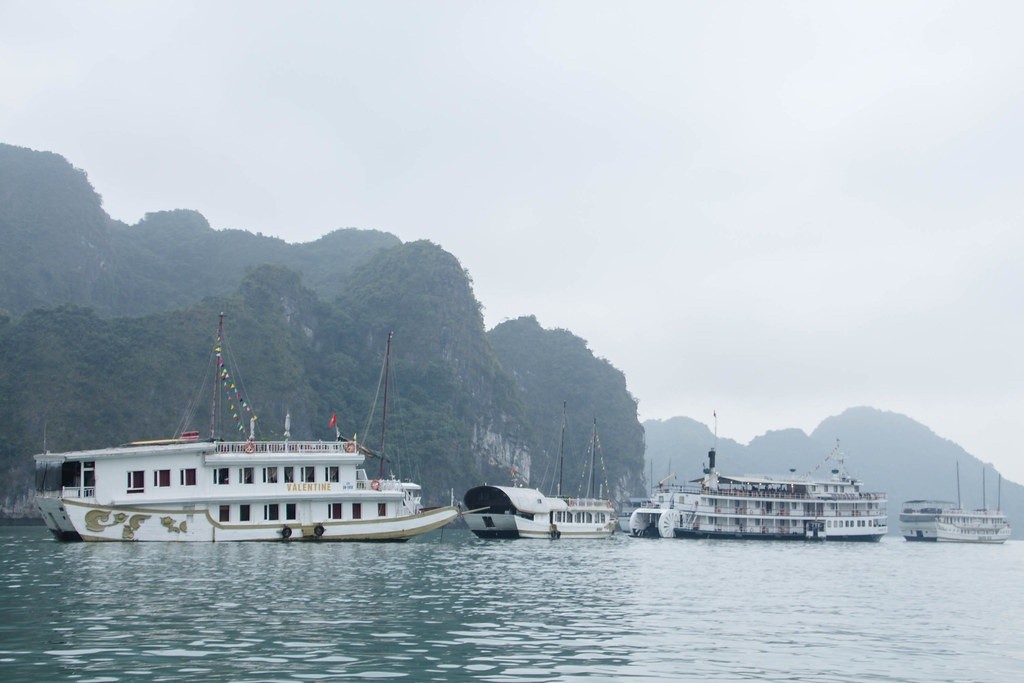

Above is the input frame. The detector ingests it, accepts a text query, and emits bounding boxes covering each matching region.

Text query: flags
[329,412,336,428]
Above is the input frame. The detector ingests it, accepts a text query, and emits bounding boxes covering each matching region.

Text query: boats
[32,311,491,542]
[899,461,1012,542]
[617,456,672,533]
[460,399,618,540]
[629,409,888,539]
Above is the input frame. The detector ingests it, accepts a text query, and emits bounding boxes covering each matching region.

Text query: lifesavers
[244,441,255,453]
[345,441,356,453]
[370,480,380,490]
